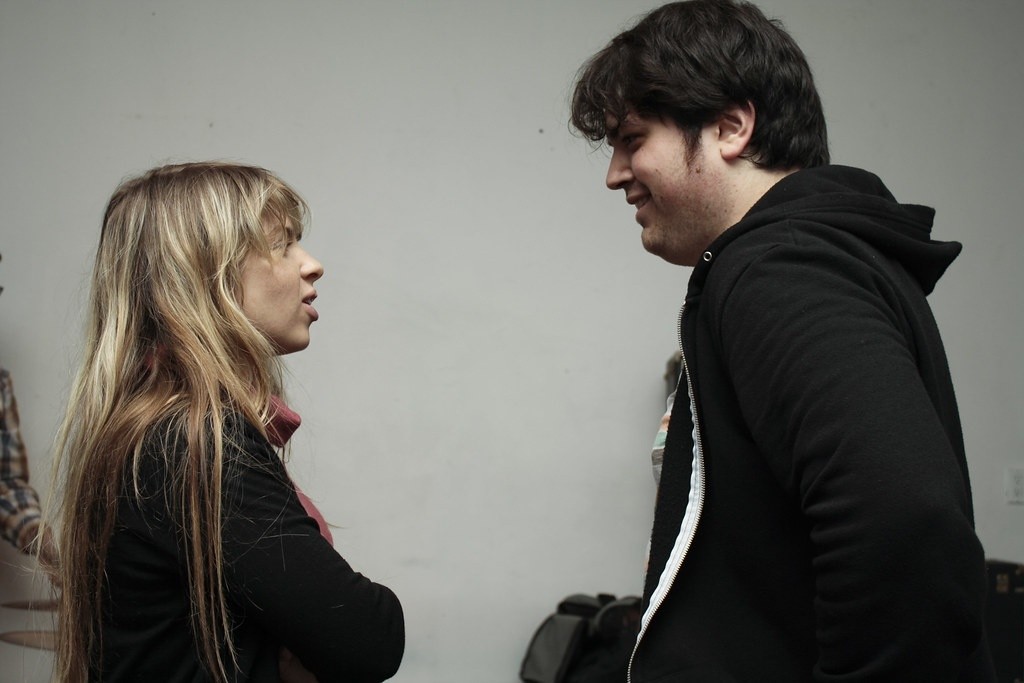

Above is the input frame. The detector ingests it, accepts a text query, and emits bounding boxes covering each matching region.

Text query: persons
[55,161,404,683]
[0,369,61,567]
[568,0,999,683]
